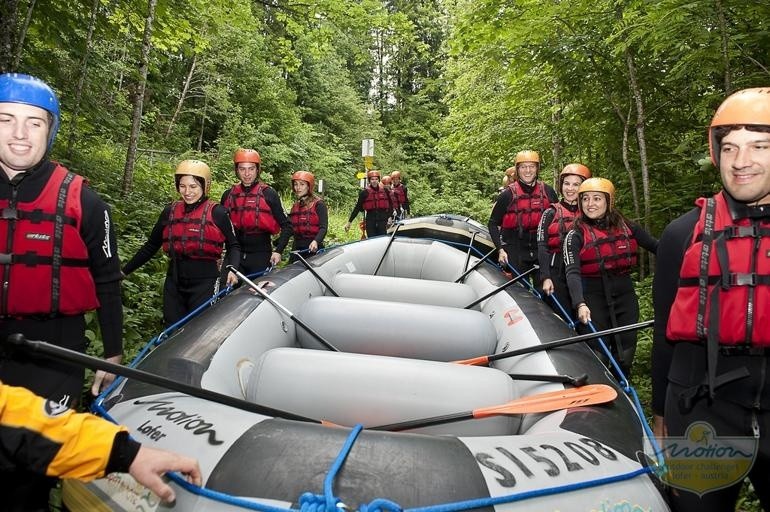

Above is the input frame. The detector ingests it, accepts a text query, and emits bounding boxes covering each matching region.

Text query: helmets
[515,150,540,179]
[368,171,380,183]
[291,171,315,194]
[504,165,516,181]
[0,73,60,152]
[392,171,400,180]
[234,148,261,180]
[175,160,211,196]
[382,176,391,185]
[559,163,591,196]
[708,87,770,168]
[578,177,615,213]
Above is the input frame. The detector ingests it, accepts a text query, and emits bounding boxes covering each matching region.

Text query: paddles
[363,383,618,431]
[452,319,656,366]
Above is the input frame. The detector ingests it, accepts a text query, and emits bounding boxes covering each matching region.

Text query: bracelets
[578,305,586,308]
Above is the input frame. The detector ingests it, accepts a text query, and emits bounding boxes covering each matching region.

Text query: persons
[2,71,125,511]
[1,380,204,503]
[217,149,292,300]
[344,170,393,238]
[488,150,559,298]
[270,171,329,265]
[536,163,593,325]
[390,171,411,220]
[380,176,402,229]
[650,86,769,512]
[119,159,242,329]
[562,177,660,386]
